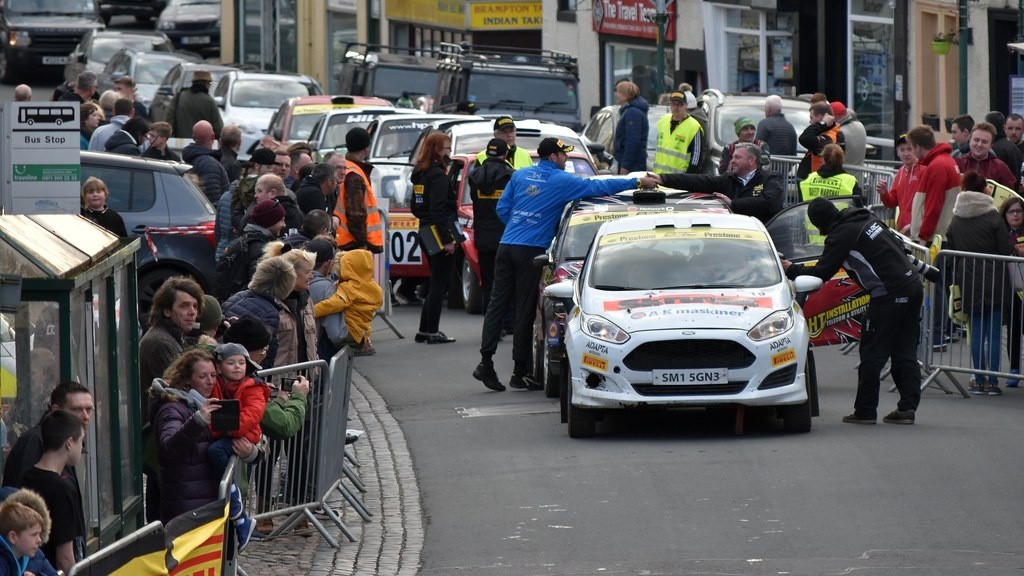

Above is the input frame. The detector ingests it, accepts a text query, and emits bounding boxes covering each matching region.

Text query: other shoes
[841,323,1019,424]
[256,518,274,533]
[292,521,312,536]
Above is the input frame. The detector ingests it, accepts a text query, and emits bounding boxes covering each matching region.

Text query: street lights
[643,0,676,105]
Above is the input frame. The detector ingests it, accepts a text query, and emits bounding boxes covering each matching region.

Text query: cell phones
[211,399,239,414]
[281,377,300,391]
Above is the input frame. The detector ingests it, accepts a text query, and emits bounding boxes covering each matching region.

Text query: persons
[780,194,925,425]
[0,38,1023,574]
[473,134,660,394]
[408,132,461,344]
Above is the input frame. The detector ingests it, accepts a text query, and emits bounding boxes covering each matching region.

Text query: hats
[194,294,223,330]
[191,71,212,82]
[537,137,575,157]
[670,90,686,104]
[250,200,285,229]
[223,315,271,352]
[345,127,371,153]
[308,238,335,262]
[213,344,263,374]
[807,197,840,226]
[830,102,847,115]
[253,147,275,164]
[894,133,908,148]
[486,138,513,157]
[733,116,756,136]
[494,115,515,130]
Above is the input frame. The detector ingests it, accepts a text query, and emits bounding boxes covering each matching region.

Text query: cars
[60,0,905,400]
[541,211,825,439]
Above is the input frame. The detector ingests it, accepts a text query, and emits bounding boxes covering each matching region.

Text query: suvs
[0,0,106,86]
[340,40,445,108]
[435,40,585,134]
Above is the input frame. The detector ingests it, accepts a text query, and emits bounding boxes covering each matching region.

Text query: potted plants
[929,29,959,55]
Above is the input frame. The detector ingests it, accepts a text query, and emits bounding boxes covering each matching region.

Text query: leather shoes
[414,331,456,344]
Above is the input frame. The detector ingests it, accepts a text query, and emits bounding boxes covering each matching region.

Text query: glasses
[1007,208,1024,214]
[332,165,348,171]
[444,146,451,151]
[271,200,281,209]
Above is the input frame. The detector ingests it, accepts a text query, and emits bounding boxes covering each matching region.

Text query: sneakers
[509,374,544,391]
[472,361,506,392]
[228,487,243,520]
[232,516,256,554]
